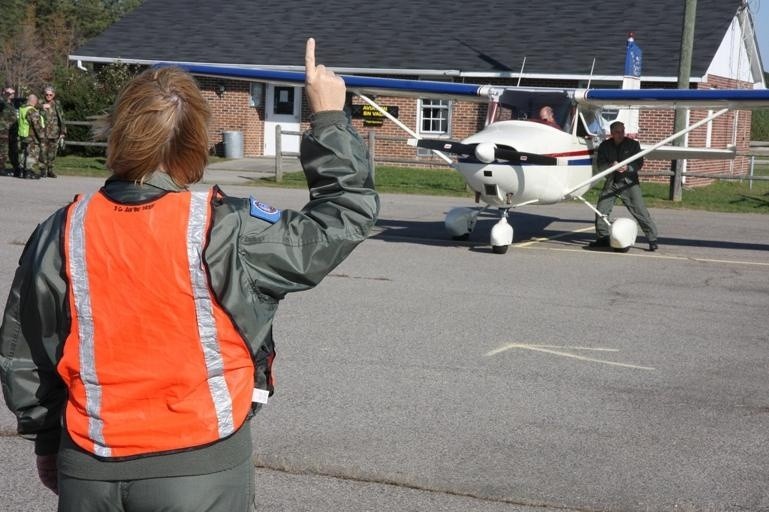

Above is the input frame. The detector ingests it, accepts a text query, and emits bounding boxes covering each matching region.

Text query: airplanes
[64,31,769,255]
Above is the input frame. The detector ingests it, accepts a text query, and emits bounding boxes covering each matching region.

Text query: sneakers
[0,170,57,179]
[649,240,658,251]
[590,238,611,248]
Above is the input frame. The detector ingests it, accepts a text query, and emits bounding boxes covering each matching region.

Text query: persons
[16,94,47,179]
[39,87,68,178]
[0,87,20,177]
[594,121,659,251]
[0,36,378,512]
[529,106,562,129]
